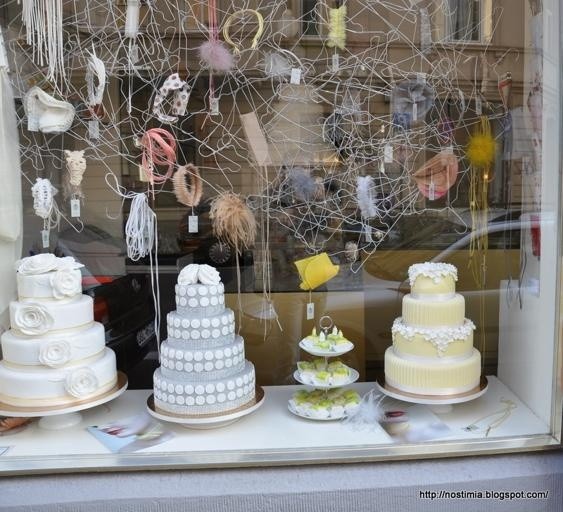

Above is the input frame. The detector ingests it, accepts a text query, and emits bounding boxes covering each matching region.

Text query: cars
[222,204,544,385]
[18,206,166,370]
[178,181,401,268]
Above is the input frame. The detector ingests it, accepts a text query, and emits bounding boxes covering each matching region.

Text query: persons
[260,146,346,383]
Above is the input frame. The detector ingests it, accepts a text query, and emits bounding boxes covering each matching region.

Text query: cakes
[287,326,361,419]
[152,263,256,416]
[0,253,120,407]
[382,263,481,396]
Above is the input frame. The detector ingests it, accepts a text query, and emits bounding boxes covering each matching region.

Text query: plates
[297,337,357,356]
[287,393,364,420]
[292,364,361,389]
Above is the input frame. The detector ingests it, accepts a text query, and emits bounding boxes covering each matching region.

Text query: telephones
[179,194,254,267]
